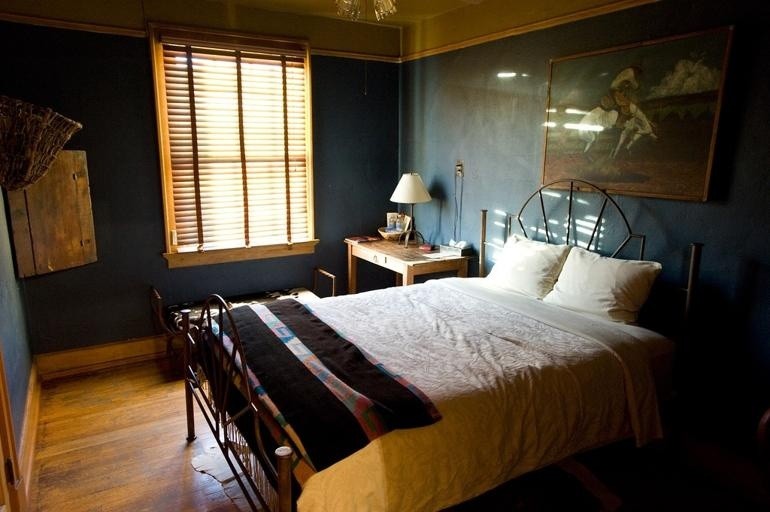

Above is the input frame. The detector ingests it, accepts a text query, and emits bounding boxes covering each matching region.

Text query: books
[345,236,383,244]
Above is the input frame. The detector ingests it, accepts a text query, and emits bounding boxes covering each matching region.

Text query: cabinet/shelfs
[5,150,99,280]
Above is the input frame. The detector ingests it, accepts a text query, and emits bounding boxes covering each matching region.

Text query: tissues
[439,238,474,257]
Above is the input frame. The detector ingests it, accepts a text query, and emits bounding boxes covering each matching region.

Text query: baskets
[378,227,405,240]
[0,95,83,192]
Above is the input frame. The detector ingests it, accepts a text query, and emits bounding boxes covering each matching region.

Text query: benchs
[151,265,339,362]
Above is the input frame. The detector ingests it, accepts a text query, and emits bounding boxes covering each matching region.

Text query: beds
[177,181,707,512]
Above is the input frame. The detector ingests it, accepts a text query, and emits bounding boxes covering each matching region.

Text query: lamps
[334,0,399,25]
[389,171,432,249]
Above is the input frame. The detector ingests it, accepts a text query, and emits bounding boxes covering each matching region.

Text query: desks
[343,234,473,294]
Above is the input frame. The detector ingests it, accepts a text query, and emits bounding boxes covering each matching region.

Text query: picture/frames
[541,25,737,204]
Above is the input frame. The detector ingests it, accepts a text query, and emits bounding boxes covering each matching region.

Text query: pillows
[484,234,570,300]
[542,245,665,327]
